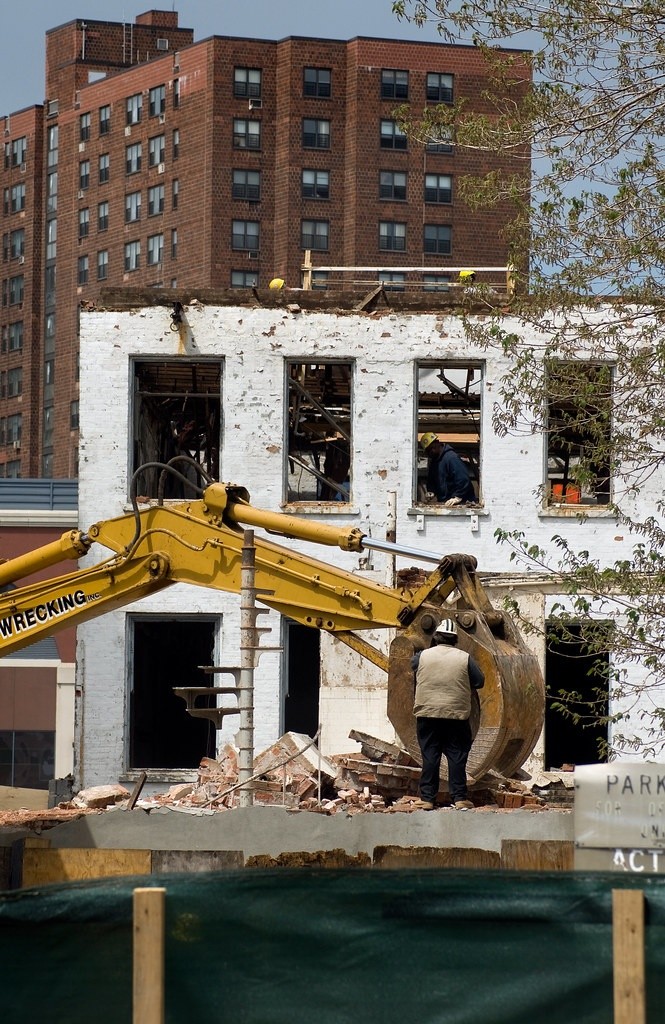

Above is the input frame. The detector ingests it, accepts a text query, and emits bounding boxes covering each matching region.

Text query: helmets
[420,431,438,451]
[436,618,458,636]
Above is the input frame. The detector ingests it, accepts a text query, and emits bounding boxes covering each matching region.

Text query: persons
[420,432,475,508]
[411,619,485,810]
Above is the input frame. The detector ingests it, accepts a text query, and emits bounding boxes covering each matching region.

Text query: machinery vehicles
[0,456,546,792]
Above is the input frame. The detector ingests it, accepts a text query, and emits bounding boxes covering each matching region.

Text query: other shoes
[455,800,474,809]
[414,799,433,809]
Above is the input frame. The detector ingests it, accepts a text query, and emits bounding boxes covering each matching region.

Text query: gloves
[445,496,462,507]
[425,492,434,498]
